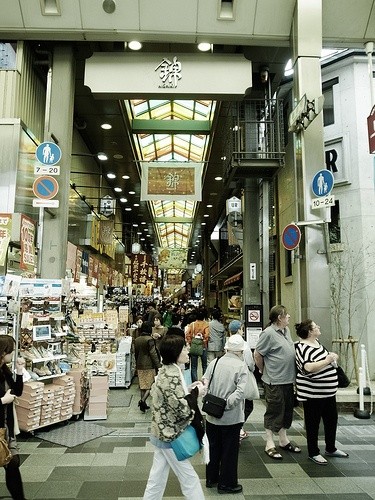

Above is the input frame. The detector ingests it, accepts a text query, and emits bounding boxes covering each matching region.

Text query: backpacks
[188,322,209,355]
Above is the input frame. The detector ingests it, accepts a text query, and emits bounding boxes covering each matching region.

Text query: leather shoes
[217,484,242,494]
[206,478,219,488]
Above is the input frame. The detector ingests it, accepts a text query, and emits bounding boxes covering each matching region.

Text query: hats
[229,320,245,330]
[225,334,247,351]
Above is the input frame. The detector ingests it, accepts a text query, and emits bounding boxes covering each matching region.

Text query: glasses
[311,323,319,331]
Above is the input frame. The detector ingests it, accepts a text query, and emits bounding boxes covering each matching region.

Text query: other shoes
[240,431,249,440]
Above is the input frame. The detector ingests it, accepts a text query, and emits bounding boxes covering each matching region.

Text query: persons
[229,320,256,441]
[0,334,32,500]
[151,314,166,374]
[184,312,210,383]
[295,319,349,464]
[166,314,186,373]
[143,335,207,500]
[207,312,225,366]
[201,334,248,493]
[134,321,161,412]
[254,304,301,459]
[132,298,220,331]
[132,316,147,375]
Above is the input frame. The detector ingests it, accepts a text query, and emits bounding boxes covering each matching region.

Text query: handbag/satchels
[171,426,201,461]
[244,372,260,400]
[0,428,12,466]
[336,365,350,388]
[202,394,226,419]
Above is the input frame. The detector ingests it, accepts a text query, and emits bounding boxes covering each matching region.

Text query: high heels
[138,400,146,413]
[144,402,150,409]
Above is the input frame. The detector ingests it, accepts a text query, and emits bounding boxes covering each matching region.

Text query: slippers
[325,451,349,457]
[265,448,282,459]
[279,443,301,453]
[310,455,328,465]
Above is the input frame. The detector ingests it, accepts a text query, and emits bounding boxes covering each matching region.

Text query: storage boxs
[13,345,127,432]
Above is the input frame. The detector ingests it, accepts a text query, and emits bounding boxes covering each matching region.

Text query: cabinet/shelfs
[0,272,71,382]
